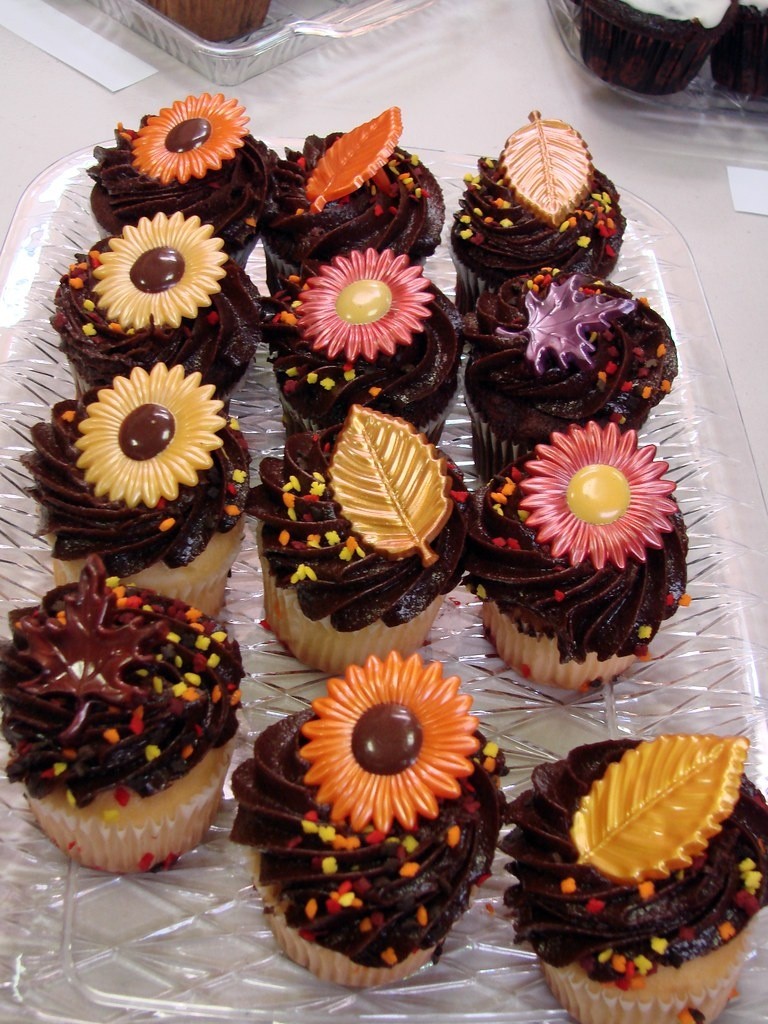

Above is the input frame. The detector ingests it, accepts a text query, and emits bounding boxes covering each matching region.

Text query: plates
[548,0,768,133]
[0,135,768,1024]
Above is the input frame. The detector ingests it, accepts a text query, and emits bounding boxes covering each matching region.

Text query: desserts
[1,0,768,1024]
